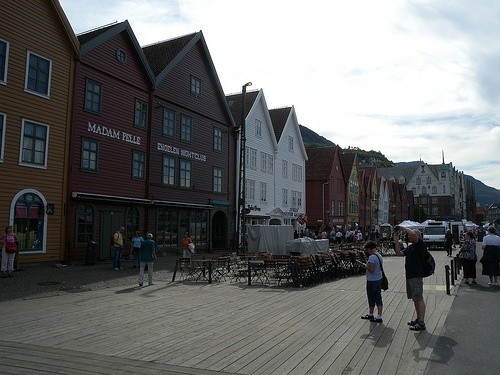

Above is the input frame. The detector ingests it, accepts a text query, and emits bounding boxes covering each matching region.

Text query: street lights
[322,182,329,224]
[370,199,377,232]
[239,82,253,257]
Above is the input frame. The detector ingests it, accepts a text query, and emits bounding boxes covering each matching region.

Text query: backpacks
[110,232,119,246]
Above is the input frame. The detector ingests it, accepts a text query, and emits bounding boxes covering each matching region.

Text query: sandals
[410,322,426,330]
[408,319,418,326]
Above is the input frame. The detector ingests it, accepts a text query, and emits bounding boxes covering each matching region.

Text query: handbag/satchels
[421,253,435,277]
[5,242,17,253]
[189,243,195,253]
[380,275,388,291]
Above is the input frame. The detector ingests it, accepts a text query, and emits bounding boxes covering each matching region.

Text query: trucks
[423,221,450,251]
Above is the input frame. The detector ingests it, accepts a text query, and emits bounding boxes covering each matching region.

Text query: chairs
[177,249,368,288]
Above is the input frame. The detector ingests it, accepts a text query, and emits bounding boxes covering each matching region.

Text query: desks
[191,259,214,266]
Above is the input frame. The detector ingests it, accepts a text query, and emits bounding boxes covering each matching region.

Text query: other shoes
[361,314,374,319]
[464,281,469,284]
[488,283,498,287]
[472,281,477,284]
[112,267,124,271]
[370,317,382,323]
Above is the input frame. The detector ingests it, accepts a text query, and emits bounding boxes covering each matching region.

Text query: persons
[132,231,144,269]
[1,226,18,278]
[361,241,383,323]
[182,232,191,267]
[443,229,454,257]
[481,225,500,287]
[139,233,155,287]
[294,228,380,243]
[392,226,426,330]
[113,226,124,270]
[461,232,477,285]
